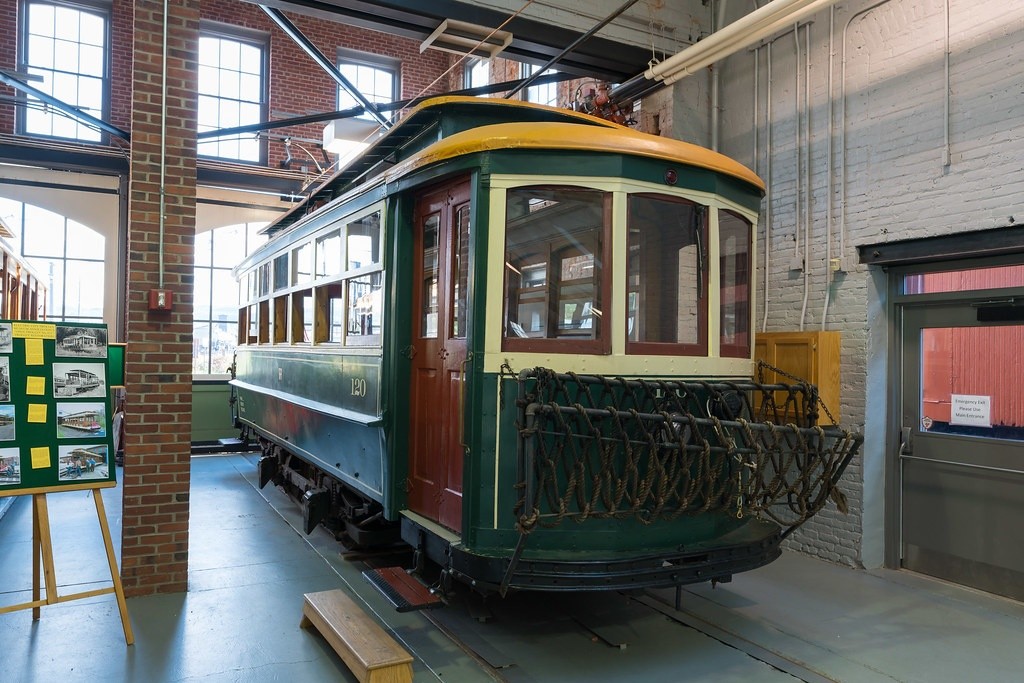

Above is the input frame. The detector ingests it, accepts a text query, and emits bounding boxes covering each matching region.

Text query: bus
[226,0,864,624]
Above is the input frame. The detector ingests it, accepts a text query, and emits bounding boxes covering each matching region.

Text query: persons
[6,466,13,477]
[75,457,82,476]
[85,459,91,473]
[66,460,74,477]
[90,458,95,472]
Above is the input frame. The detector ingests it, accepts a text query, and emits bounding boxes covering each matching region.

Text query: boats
[61,410,101,433]
[67,449,103,470]
[64,369,100,392]
[61,336,98,352]
